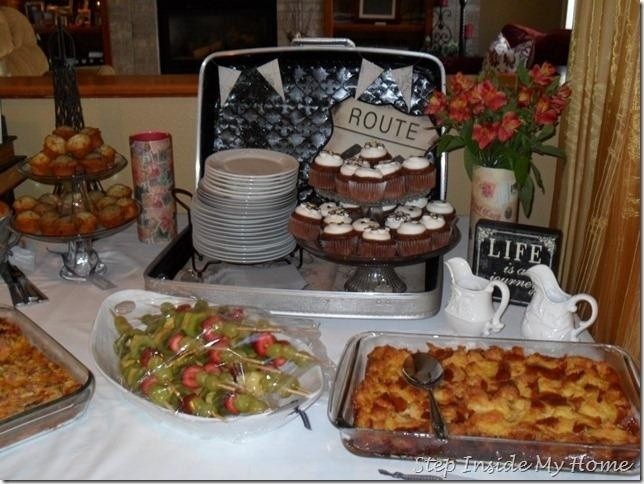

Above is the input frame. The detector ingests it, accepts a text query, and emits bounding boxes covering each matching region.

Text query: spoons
[401,353,448,441]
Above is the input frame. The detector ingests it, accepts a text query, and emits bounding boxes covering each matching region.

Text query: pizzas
[0,316,84,423]
[349,343,640,467]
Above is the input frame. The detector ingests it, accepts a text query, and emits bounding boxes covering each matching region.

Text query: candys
[113,301,310,418]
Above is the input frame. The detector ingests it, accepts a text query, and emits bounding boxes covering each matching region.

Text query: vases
[464,165,521,268]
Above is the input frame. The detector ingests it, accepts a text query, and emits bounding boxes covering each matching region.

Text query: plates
[327,330,641,476]
[0,304,94,452]
[90,289,324,440]
[191,149,298,265]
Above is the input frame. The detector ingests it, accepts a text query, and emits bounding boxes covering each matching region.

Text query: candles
[465,23,474,39]
[439,0,449,7]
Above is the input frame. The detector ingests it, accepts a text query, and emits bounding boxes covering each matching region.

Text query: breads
[30,125,116,178]
[14,183,137,237]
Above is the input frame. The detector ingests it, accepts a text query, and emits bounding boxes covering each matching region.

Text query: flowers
[423,61,572,219]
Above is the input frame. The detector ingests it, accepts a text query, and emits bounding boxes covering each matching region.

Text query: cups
[445,257,598,351]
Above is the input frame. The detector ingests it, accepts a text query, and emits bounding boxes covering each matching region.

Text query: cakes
[319,202,337,219]
[385,213,411,239]
[339,201,363,223]
[322,222,355,256]
[363,203,397,219]
[405,196,428,213]
[337,156,360,178]
[374,156,405,179]
[397,219,431,257]
[427,198,459,239]
[360,226,395,258]
[308,151,344,170]
[395,202,424,221]
[323,208,352,229]
[402,155,436,172]
[349,166,385,182]
[420,212,451,248]
[354,217,381,242]
[289,200,321,218]
[357,142,389,165]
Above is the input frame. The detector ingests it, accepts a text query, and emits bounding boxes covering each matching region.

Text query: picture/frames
[352,0,402,24]
